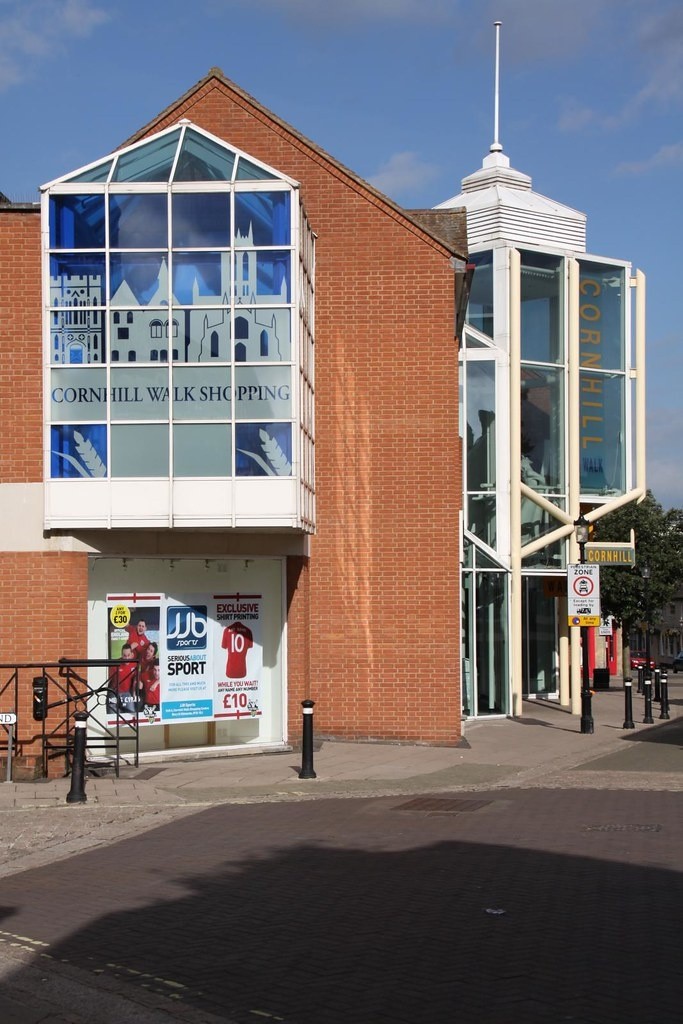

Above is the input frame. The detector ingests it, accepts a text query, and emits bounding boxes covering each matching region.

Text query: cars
[630,650,655,671]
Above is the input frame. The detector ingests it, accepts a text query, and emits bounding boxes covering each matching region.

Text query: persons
[108,619,160,713]
